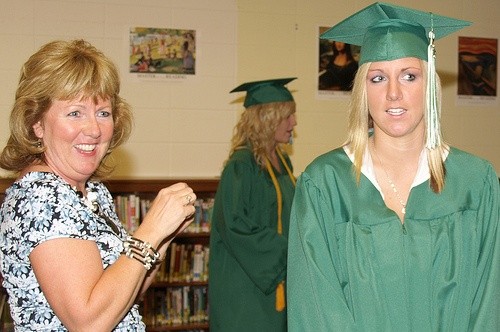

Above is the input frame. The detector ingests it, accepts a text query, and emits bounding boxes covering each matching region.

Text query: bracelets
[120,235,160,270]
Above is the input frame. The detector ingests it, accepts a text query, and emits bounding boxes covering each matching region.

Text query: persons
[0,39,197,332]
[207,77,297,332]
[287,2,500,332]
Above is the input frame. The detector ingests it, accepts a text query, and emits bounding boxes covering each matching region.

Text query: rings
[185,195,191,205]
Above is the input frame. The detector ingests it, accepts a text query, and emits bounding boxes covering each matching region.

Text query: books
[135,284,210,325]
[0,294,14,332]
[154,242,210,282]
[113,193,215,233]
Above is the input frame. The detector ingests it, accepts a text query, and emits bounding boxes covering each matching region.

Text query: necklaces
[373,143,406,213]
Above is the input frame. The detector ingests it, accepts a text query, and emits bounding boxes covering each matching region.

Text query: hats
[229,76,298,107]
[319,1,473,65]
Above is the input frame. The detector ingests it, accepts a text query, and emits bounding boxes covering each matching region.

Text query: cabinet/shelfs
[1,176,221,331]
